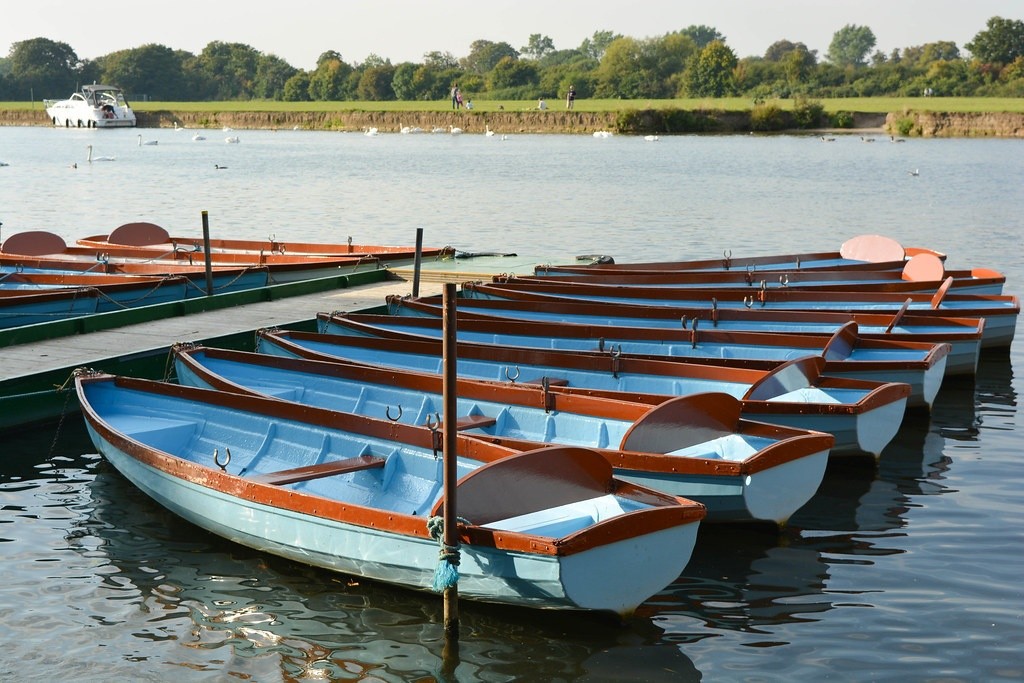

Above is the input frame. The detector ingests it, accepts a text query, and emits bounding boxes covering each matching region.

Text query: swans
[905,167,921,178]
[819,134,907,148]
[362,122,660,142]
[86,121,301,164]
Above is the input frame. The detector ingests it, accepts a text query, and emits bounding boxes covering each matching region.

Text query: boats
[47,85,138,129]
[1,221,1021,619]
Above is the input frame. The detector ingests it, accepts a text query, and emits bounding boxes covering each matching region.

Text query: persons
[450,83,464,109]
[538,97,548,111]
[466,101,473,109]
[567,86,577,109]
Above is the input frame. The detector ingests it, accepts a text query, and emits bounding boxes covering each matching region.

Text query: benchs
[243,454,385,487]
[523,377,570,387]
[415,413,497,432]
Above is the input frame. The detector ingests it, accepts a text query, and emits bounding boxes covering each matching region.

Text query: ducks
[214,164,229,170]
[67,164,79,169]
[0,161,10,169]
